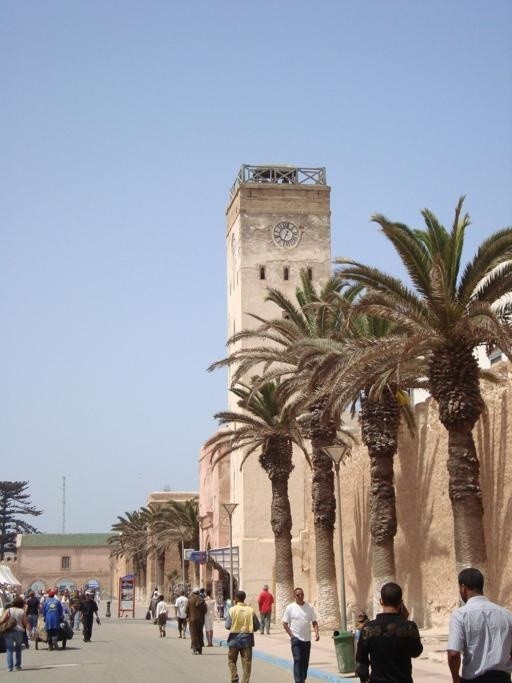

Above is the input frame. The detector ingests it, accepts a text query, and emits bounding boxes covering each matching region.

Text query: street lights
[223,502,238,609]
[321,443,350,630]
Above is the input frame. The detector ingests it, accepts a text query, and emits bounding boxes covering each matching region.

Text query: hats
[84,591,94,594]
[193,588,200,593]
[49,592,54,596]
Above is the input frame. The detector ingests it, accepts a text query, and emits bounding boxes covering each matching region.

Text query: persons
[357,581,423,682]
[0,597,31,672]
[256,584,275,636]
[153,594,169,637]
[281,588,321,683]
[203,587,217,647]
[26,585,101,648]
[183,588,208,655]
[151,587,160,601]
[147,591,161,625]
[223,592,233,631]
[174,590,189,639]
[199,588,206,598]
[355,607,370,678]
[445,567,512,682]
[224,590,260,682]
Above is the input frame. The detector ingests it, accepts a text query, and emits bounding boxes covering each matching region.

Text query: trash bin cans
[331,630,356,673]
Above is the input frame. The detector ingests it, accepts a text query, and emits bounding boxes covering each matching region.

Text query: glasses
[296,594,304,597]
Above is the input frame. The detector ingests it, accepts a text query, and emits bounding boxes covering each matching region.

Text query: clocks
[273,220,301,251]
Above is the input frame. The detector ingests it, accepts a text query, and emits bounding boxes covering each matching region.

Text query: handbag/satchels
[146,612,151,620]
[0,617,17,634]
